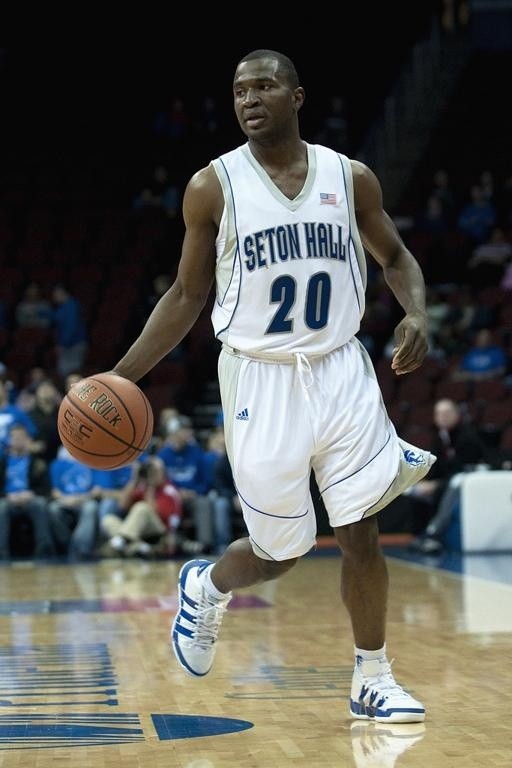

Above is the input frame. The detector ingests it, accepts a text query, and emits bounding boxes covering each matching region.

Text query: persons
[1,1,511,562]
[67,46,439,727]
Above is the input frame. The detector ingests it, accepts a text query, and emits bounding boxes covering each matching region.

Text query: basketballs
[57,370,153,469]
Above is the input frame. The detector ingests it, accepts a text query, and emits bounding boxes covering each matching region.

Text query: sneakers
[170,558,232,678]
[348,656,427,724]
